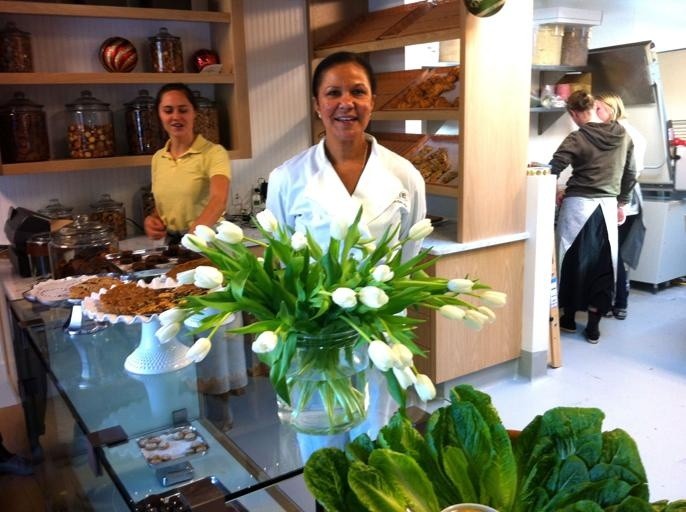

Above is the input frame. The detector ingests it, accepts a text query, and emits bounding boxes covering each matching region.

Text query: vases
[271,326,373,439]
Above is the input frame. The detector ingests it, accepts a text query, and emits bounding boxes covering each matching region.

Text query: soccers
[99,36,138,71]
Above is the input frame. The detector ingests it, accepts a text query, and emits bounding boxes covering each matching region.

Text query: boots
[583,308,601,343]
[560,309,577,333]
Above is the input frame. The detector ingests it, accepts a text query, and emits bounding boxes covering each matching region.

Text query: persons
[143,83,230,244]
[548,88,646,344]
[263,51,427,512]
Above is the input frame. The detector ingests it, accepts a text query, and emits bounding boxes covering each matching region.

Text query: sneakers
[602,308,627,320]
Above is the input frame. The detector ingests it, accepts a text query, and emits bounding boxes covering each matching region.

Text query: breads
[69,276,124,297]
[120,244,203,271]
[99,284,157,314]
[411,145,458,183]
[395,67,460,110]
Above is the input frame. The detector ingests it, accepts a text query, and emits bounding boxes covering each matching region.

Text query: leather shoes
[0,457,33,475]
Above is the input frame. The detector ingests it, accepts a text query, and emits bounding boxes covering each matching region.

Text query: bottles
[190,91,221,144]
[147,27,185,73]
[27,193,127,280]
[0,90,51,164]
[62,89,116,158]
[123,89,164,155]
[0,21,34,72]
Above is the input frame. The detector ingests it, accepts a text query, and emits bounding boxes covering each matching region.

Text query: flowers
[147,202,513,403]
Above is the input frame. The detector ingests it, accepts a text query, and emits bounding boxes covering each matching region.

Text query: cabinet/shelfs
[530,65,599,113]
[304,0,530,397]
[1,0,254,174]
